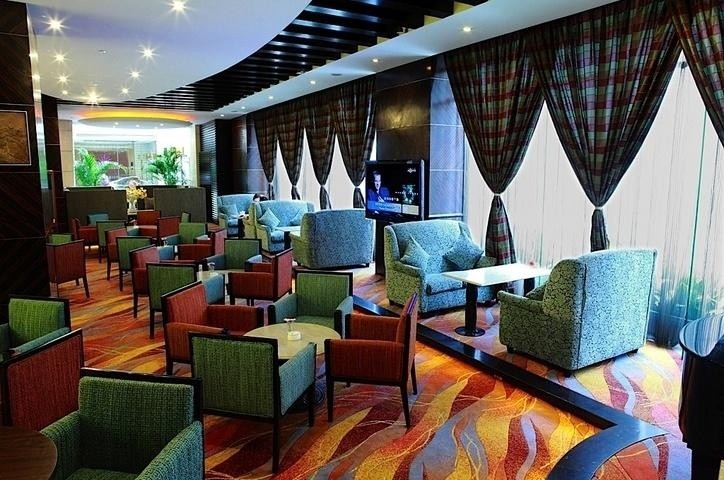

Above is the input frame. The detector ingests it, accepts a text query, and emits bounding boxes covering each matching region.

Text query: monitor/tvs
[365,159,425,224]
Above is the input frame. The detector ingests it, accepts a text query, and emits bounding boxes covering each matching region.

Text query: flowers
[126,188,147,205]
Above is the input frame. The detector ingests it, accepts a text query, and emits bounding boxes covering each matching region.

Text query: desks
[439,263,553,337]
[244,322,341,412]
[0,426,59,480]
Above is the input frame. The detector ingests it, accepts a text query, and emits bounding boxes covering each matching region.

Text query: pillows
[526,281,545,301]
[443,232,485,270]
[400,236,430,271]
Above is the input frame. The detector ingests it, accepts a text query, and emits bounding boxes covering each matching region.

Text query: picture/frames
[0,103,40,173]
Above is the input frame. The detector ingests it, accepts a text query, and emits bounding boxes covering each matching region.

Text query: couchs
[216,194,375,269]
[497,248,659,378]
[384,220,499,319]
[136,185,206,223]
[64,186,128,221]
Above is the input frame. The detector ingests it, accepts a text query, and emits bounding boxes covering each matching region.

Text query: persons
[367,170,392,214]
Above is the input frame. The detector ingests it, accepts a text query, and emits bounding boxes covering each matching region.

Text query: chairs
[323,293,419,426]
[0,297,85,431]
[187,331,317,472]
[38,367,205,479]
[46,210,353,378]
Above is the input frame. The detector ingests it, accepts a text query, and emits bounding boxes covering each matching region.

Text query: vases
[128,200,137,212]
